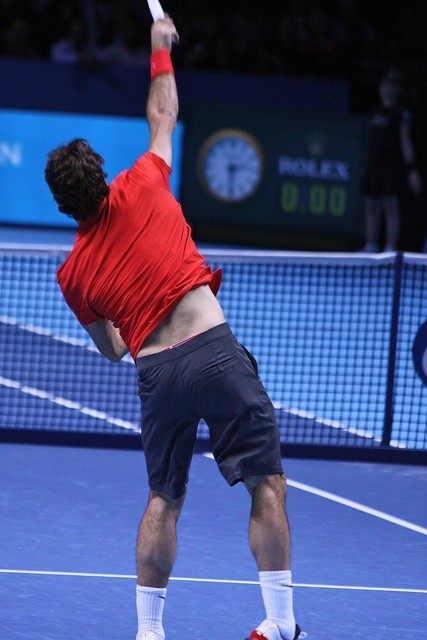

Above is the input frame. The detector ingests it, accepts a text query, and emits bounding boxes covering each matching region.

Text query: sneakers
[244,619,307,639]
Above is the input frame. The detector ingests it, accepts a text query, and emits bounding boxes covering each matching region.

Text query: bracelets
[149,47,176,80]
[406,161,418,172]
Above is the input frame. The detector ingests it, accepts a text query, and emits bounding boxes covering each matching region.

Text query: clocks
[198,131,262,200]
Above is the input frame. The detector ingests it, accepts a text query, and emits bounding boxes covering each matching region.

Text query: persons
[0,0,427,78]
[358,75,424,253]
[44,14,307,640]
